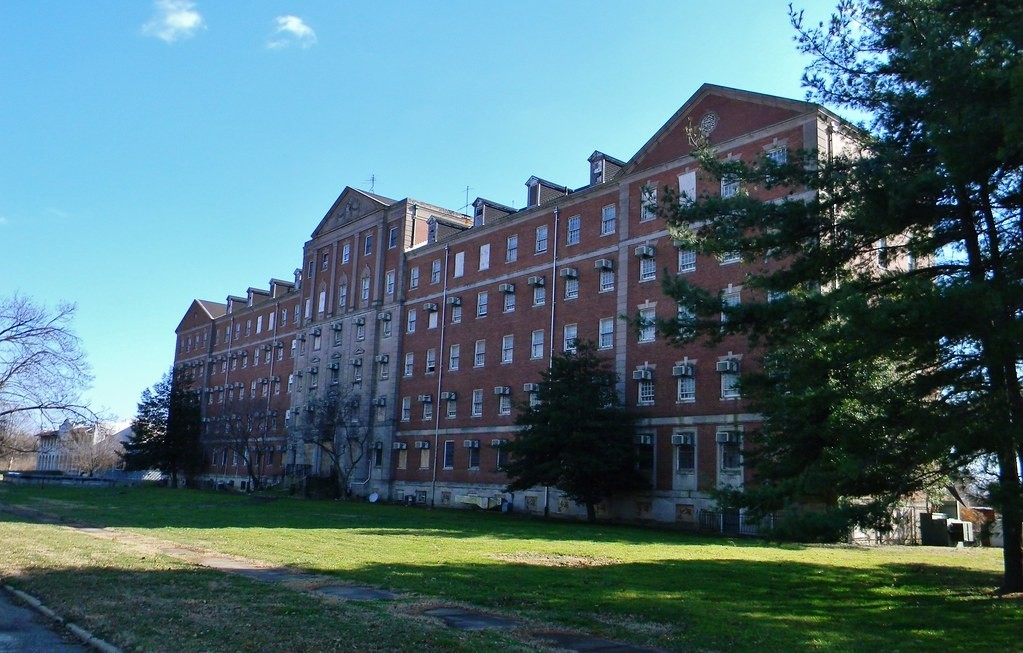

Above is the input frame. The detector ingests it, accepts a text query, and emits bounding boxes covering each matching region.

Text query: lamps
[635,245,654,257]
[440,391,455,402]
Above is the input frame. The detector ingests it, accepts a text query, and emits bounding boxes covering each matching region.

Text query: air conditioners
[631,368,652,380]
[492,438,508,447]
[560,267,576,277]
[631,436,650,444]
[672,365,693,375]
[716,430,736,443]
[673,239,681,247]
[415,440,429,449]
[417,394,432,402]
[463,439,479,448]
[595,257,613,269]
[177,312,390,449]
[424,302,437,312]
[499,283,513,292]
[669,435,690,446]
[527,275,545,285]
[524,381,543,392]
[495,385,511,395]
[448,296,460,305]
[392,443,406,450]
[716,360,739,371]
